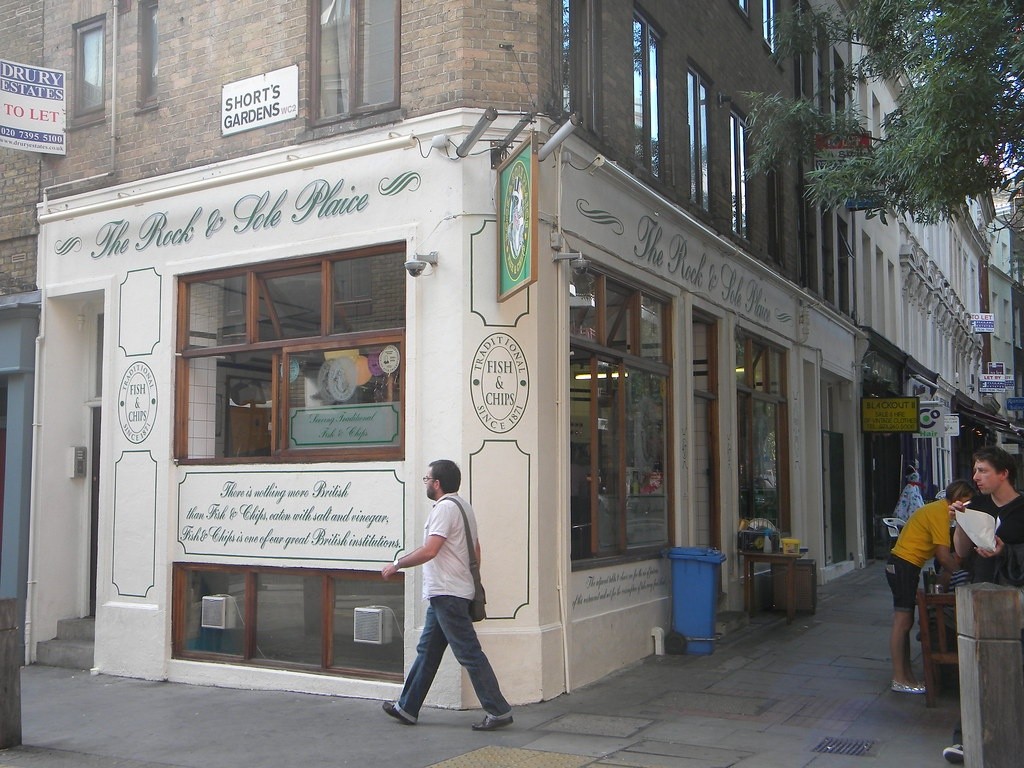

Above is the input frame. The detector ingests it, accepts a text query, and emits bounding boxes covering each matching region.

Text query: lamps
[538,110,582,163]
[403,251,438,278]
[456,103,498,160]
[551,250,592,274]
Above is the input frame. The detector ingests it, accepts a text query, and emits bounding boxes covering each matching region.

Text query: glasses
[423,477,441,484]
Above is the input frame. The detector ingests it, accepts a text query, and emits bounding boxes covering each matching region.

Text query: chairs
[917,588,958,708]
[882,517,906,552]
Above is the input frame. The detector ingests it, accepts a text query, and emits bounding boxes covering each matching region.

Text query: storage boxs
[770,559,819,617]
[715,610,748,637]
[781,538,800,555]
[738,529,781,555]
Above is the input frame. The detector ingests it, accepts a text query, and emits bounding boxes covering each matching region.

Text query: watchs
[392,560,400,570]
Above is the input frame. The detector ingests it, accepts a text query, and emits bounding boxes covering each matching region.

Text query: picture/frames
[224,374,281,458]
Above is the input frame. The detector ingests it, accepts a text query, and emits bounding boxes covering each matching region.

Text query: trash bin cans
[662,546,728,657]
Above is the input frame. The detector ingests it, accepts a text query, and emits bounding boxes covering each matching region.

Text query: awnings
[960,403,1024,444]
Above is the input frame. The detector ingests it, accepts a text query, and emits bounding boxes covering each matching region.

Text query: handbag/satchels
[466,568,487,622]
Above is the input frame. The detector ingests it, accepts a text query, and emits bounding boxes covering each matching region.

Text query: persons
[382,459,514,729]
[885,479,974,692]
[942,448,1024,763]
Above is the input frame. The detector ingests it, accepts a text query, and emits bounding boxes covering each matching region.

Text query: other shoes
[383,700,413,724]
[471,711,513,732]
[942,743,964,763]
[892,675,926,694]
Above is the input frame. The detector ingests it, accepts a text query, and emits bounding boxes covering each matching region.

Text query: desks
[737,550,807,625]
